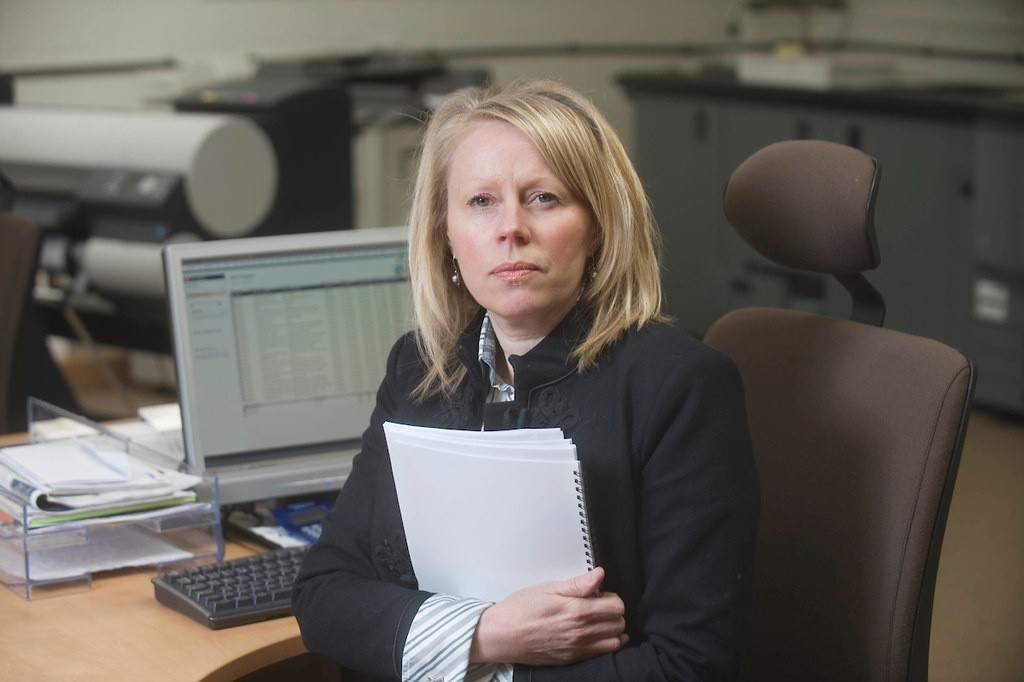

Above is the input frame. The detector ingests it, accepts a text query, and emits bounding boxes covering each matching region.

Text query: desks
[0,414,332,681]
[620,60,1022,413]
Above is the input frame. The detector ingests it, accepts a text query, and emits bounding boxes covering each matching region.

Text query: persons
[290,75,762,682]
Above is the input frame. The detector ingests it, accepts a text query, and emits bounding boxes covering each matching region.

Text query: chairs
[297,139,982,679]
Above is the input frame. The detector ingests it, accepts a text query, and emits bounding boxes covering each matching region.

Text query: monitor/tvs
[160,226,420,553]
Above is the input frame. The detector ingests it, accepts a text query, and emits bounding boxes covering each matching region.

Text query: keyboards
[150,544,312,632]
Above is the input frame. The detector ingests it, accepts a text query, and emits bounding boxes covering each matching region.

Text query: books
[1,438,211,530]
[379,420,601,606]
